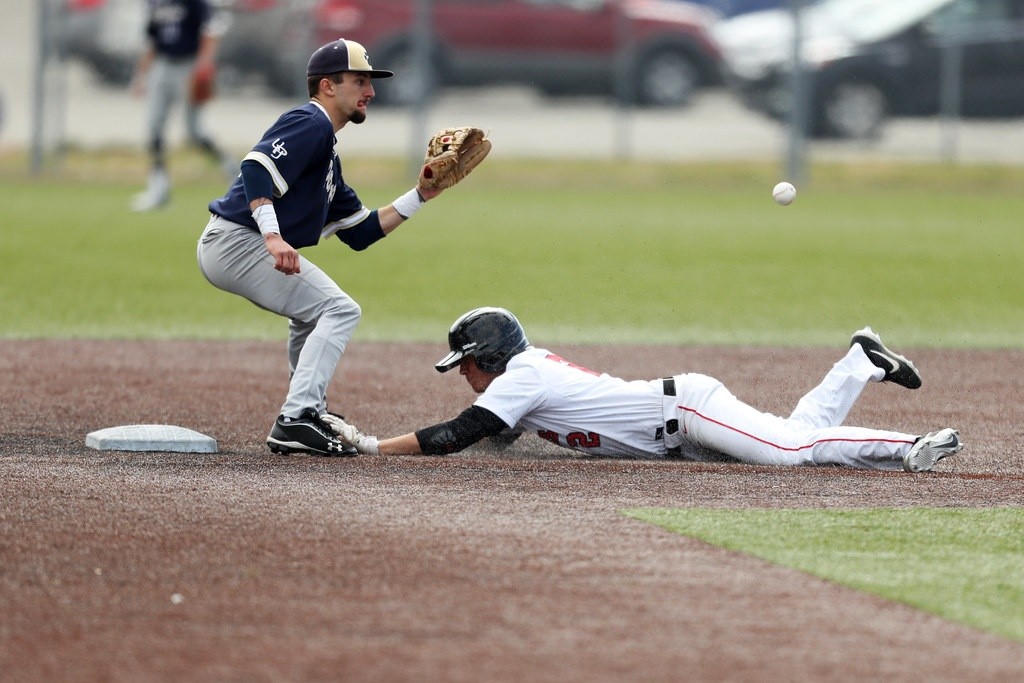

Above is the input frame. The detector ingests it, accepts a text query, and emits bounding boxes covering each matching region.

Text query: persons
[130,1,238,213]
[195,38,491,462]
[318,307,966,474]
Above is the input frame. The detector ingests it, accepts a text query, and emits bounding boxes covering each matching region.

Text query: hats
[306,37,394,78]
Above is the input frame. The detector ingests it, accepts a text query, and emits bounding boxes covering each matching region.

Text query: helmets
[435,307,530,373]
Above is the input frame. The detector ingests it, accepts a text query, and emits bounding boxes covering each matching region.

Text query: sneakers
[266,415,359,458]
[850,326,922,390]
[318,409,352,425]
[903,428,964,472]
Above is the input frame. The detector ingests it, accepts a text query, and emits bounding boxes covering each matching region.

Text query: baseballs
[772,181,798,206]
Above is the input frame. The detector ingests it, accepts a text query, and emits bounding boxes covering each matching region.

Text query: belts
[662,377,682,458]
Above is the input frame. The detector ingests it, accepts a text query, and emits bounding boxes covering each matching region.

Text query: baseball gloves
[417,126,493,202]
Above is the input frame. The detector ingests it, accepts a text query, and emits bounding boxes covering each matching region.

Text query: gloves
[320,413,379,455]
